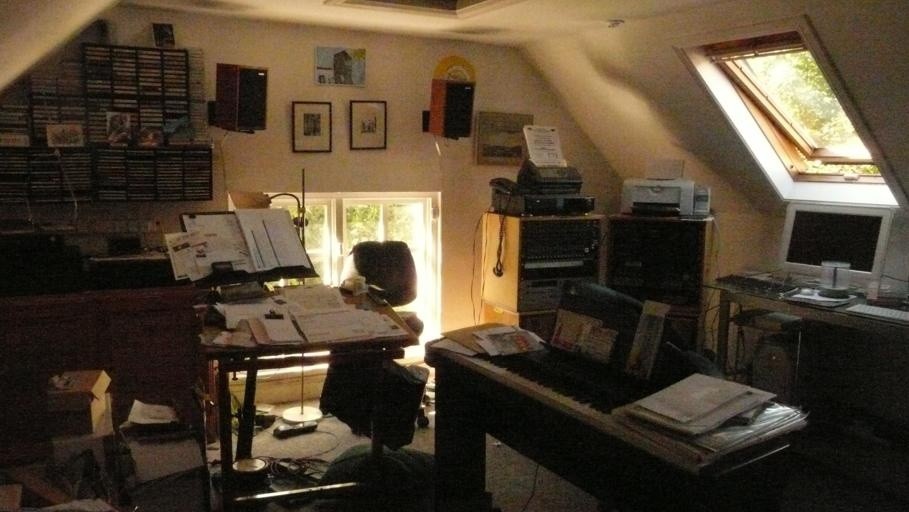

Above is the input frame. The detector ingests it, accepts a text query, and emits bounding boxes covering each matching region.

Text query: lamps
[228,163,325,430]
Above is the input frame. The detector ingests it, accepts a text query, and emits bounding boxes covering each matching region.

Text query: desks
[706,270,909,390]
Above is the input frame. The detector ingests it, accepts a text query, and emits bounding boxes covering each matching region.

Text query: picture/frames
[314,45,368,88]
[292,101,332,153]
[47,122,86,146]
[475,110,536,167]
[350,99,387,150]
[105,110,132,146]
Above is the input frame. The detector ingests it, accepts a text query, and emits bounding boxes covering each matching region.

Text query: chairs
[348,240,432,435]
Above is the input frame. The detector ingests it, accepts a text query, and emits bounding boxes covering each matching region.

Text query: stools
[730,309,810,410]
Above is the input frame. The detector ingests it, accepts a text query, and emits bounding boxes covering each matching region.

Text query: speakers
[215,62,269,132]
[430,76,476,140]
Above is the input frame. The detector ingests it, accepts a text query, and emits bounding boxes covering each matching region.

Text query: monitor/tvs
[781,201,898,305]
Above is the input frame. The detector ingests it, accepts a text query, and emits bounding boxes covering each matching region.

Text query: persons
[107,112,132,147]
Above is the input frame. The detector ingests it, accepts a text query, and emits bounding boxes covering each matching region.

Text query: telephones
[489,178,524,195]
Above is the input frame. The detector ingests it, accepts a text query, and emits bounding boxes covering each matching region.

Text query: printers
[487,160,596,217]
[621,178,712,219]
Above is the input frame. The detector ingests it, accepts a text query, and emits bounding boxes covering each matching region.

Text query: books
[431,320,548,357]
[610,370,806,465]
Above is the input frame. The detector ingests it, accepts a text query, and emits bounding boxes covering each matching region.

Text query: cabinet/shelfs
[608,212,714,353]
[481,211,604,325]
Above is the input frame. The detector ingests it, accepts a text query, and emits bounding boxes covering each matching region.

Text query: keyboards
[846,304,909,325]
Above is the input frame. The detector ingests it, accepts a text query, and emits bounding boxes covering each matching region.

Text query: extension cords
[275,457,325,483]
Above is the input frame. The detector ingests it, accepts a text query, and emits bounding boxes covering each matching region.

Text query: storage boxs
[0,367,209,512]
